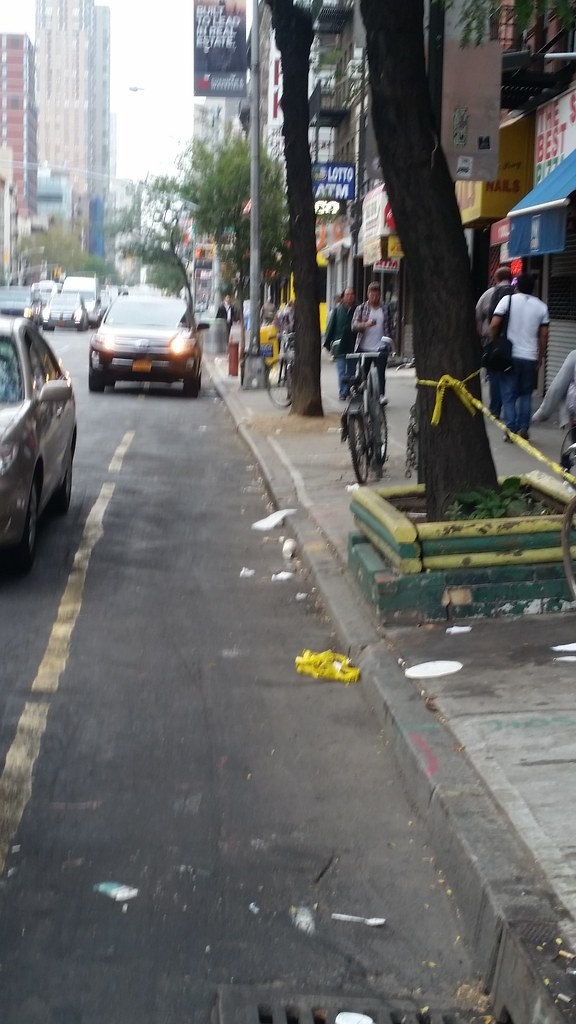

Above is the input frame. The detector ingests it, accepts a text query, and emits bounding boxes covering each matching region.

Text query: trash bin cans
[197,316,228,357]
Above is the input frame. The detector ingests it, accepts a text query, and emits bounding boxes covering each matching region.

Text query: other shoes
[379,395,389,407]
[503,429,529,445]
[339,395,346,400]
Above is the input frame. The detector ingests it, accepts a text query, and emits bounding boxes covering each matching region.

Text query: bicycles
[340,351,392,486]
[263,331,296,406]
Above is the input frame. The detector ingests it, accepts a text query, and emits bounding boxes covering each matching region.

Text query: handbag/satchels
[478,338,512,376]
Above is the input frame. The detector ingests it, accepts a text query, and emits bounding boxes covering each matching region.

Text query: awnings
[507,149,576,258]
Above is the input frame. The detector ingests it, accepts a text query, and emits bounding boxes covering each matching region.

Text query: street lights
[127,81,228,316]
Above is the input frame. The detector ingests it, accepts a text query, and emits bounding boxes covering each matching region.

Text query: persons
[531,349,576,444]
[216,294,234,331]
[476,266,549,443]
[323,282,390,407]
[242,297,251,332]
[260,296,276,326]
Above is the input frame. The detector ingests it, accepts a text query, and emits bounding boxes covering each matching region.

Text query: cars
[87,295,202,398]
[1,316,78,571]
[2,272,159,332]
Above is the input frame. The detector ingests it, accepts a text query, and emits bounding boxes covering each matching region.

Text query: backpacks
[489,285,516,329]
